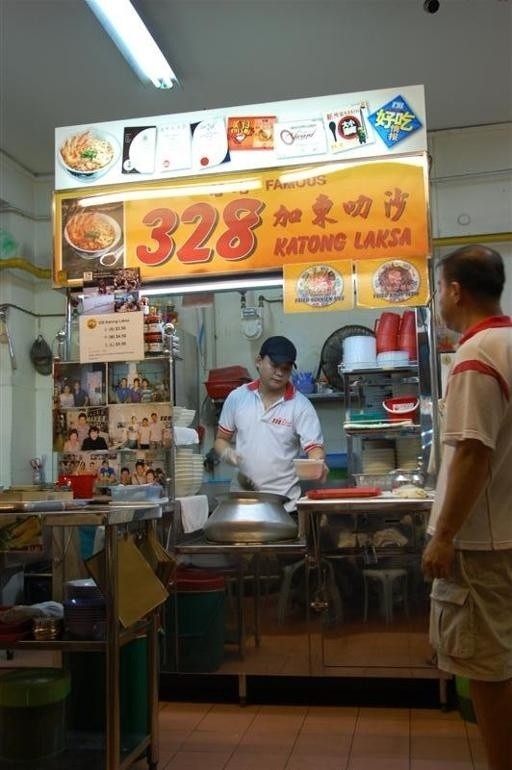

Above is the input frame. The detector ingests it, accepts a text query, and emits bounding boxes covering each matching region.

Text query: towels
[170,426,201,446]
[176,494,209,533]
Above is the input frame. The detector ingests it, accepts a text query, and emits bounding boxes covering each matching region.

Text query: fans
[315,325,375,393]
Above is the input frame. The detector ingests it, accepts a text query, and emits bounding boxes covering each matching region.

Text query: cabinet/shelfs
[335,362,425,491]
[50,334,183,679]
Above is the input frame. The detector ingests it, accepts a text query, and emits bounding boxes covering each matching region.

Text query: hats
[259,336,297,369]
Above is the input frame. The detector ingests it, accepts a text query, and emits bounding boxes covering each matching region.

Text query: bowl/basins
[203,488,300,541]
[62,580,105,636]
[171,404,205,498]
[65,210,120,254]
[57,129,120,181]
[293,457,325,482]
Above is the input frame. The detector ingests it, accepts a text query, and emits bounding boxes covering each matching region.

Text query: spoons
[99,247,124,266]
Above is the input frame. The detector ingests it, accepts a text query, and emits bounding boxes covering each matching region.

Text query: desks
[0,504,165,770]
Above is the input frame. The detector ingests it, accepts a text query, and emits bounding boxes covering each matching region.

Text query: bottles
[145,316,163,355]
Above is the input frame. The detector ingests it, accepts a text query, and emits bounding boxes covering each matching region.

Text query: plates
[295,266,344,307]
[362,436,422,474]
[372,260,420,304]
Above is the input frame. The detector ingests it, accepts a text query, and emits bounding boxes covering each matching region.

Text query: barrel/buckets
[338,336,378,372]
[119,635,152,750]
[68,474,97,498]
[0,666,67,770]
[382,394,420,424]
[174,573,225,590]
[173,590,227,673]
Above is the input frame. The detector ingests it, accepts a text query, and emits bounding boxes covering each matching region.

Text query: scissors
[30,458,41,471]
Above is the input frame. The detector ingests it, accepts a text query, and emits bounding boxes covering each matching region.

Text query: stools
[363,569,411,630]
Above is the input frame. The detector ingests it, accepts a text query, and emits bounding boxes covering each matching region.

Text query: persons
[59,381,107,449]
[79,461,155,485]
[420,244,512,770]
[111,268,139,291]
[117,378,165,449]
[213,337,329,522]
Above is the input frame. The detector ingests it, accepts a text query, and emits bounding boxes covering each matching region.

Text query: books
[84,533,176,629]
[0,491,73,502]
